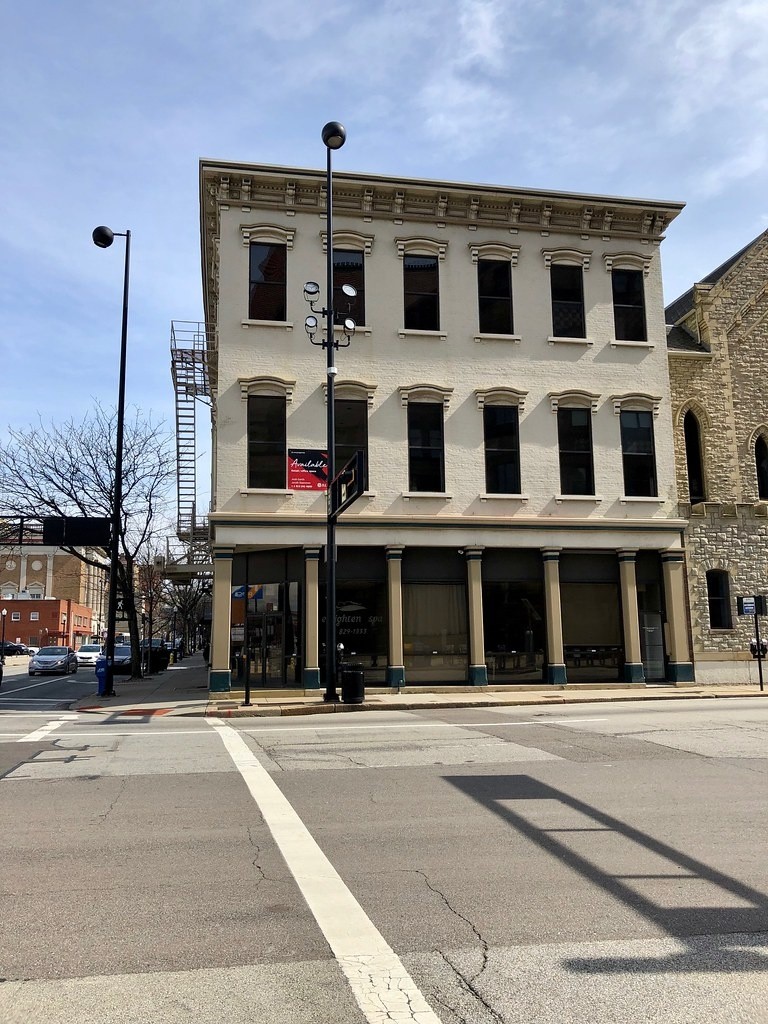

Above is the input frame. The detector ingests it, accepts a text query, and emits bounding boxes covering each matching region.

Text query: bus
[114,632,131,645]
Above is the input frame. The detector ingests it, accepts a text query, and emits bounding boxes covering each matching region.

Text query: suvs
[14,643,40,657]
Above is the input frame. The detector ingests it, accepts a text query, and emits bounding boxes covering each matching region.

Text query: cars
[74,642,106,667]
[164,642,173,650]
[28,646,78,676]
[0,641,29,655]
[94,645,132,675]
[140,637,171,664]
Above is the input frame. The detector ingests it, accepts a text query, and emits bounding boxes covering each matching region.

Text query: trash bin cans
[340,661,365,704]
[147,647,163,675]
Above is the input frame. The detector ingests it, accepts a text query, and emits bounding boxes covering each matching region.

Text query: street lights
[91,227,131,697]
[2,608,8,660]
[303,122,357,701]
[172,605,178,650]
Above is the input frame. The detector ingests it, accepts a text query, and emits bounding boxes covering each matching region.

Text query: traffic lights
[116,597,125,612]
[338,460,359,498]
